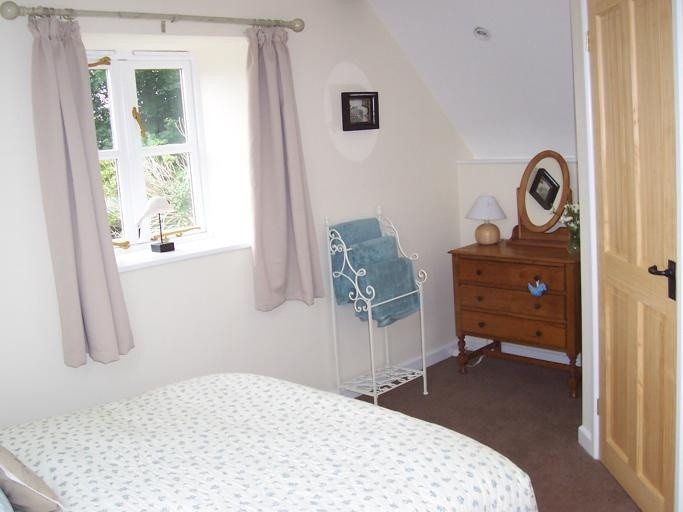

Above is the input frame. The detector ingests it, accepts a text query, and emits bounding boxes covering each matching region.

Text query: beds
[0,371,541,512]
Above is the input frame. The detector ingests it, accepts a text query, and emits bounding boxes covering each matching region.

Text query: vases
[565,229,580,255]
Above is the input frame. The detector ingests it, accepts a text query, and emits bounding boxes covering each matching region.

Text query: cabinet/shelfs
[446,238,582,398]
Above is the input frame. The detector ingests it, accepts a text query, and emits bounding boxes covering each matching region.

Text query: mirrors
[517,149,569,235]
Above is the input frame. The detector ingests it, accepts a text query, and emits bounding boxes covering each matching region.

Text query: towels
[342,234,399,322]
[327,217,381,305]
[363,257,420,329]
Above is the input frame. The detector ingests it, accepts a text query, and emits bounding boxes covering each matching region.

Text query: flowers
[550,200,580,255]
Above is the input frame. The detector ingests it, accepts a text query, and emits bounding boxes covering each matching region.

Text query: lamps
[464,193,507,245]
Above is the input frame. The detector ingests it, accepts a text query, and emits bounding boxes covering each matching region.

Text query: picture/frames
[340,91,379,131]
[528,168,559,210]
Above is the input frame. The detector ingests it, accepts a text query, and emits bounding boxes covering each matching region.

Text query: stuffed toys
[527,283,547,297]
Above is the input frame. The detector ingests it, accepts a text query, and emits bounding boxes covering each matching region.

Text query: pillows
[0,448,63,512]
